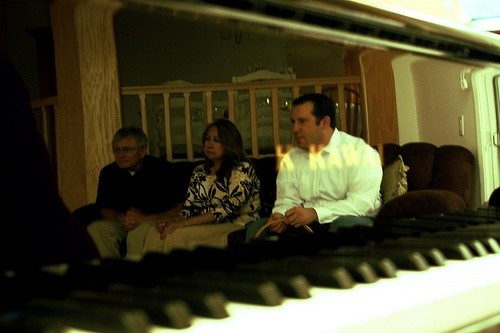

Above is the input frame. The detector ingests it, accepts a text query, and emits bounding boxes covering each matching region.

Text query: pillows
[382,153,410,205]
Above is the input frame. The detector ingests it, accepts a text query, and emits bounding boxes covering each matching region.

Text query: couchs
[70,142,475,293]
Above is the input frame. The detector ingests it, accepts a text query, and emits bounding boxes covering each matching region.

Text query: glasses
[113,145,139,153]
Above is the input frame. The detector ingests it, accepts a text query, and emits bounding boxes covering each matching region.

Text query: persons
[69,126,181,290]
[142,119,261,276]
[266,93,383,259]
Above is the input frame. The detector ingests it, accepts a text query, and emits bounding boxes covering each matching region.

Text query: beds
[153,69,299,155]
